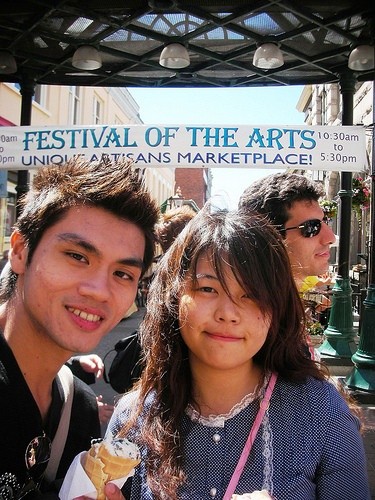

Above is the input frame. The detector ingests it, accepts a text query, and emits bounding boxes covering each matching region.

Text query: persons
[103,208,368,500]
[0,154,158,500]
[0,171,335,424]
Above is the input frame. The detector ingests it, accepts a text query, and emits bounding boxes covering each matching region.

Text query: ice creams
[84,437,142,500]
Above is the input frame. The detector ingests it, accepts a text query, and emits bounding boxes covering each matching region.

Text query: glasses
[280,216,328,237]
[18,429,52,500]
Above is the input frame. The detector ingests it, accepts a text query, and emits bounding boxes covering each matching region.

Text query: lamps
[349,46,375,70]
[253,44,284,69]
[160,43,190,68]
[72,42,102,70]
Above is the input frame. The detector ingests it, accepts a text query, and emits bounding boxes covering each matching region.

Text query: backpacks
[103,330,148,394]
